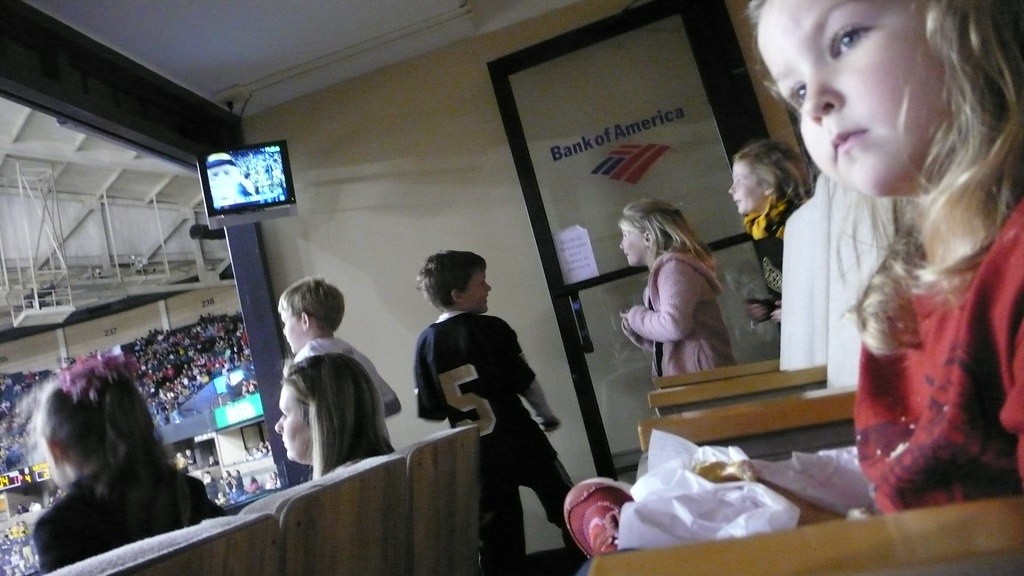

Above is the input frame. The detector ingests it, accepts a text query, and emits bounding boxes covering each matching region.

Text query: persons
[728,141,815,333]
[277,275,402,419]
[0,312,281,575]
[563,0,1024,576]
[617,199,735,381]
[30,352,227,576]
[206,147,286,209]
[414,251,587,576]
[274,353,394,481]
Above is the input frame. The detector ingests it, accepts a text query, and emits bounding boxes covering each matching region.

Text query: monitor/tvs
[196,140,299,230]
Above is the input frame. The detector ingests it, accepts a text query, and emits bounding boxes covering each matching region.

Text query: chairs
[43,423,483,576]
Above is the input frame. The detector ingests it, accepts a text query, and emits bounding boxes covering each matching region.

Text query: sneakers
[564,477,635,560]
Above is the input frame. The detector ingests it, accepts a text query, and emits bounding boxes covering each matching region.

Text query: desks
[585,360,1024,576]
